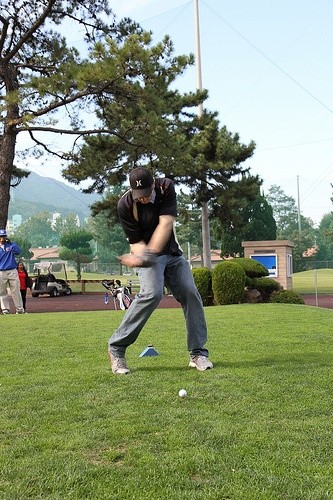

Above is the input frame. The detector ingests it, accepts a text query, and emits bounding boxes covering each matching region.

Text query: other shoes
[2,309,10,314]
[16,310,28,314]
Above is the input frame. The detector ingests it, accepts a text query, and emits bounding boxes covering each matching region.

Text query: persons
[16,262,32,314]
[0,229,24,315]
[108,167,213,374]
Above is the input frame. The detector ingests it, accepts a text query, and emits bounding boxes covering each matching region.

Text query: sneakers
[189,354,213,370]
[109,351,130,374]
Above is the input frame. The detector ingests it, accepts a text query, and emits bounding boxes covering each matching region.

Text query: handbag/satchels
[26,273,32,288]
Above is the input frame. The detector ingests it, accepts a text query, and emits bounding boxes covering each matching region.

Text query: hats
[0,229,7,236]
[129,166,154,200]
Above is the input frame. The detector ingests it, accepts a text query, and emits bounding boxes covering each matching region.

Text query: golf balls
[178,388,188,397]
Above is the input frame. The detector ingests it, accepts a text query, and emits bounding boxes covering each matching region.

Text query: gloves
[137,249,157,267]
